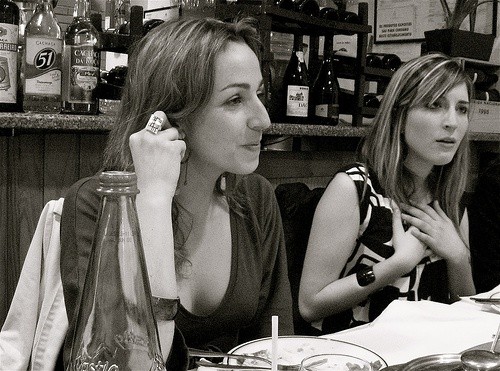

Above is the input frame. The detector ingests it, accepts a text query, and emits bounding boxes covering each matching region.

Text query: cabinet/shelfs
[215,0,398,127]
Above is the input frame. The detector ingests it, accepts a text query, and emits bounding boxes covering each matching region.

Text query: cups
[300,354,380,371]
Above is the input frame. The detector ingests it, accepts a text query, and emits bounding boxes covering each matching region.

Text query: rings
[146,115,163,135]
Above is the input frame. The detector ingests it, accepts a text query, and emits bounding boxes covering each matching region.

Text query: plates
[223,336,389,371]
[490,291,500,312]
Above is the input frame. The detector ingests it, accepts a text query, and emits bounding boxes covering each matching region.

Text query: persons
[300,53,476,334]
[55,19,295,371]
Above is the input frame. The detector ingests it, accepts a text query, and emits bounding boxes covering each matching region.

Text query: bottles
[66,171,166,371]
[281,35,311,124]
[312,36,340,126]
[22,0,64,114]
[0,0,24,113]
[237,0,359,36]
[340,88,384,118]
[334,48,402,70]
[62,0,101,115]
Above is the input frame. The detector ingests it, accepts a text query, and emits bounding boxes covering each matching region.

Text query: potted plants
[424,0,496,62]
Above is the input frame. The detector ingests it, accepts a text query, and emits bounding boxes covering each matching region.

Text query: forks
[188,348,328,369]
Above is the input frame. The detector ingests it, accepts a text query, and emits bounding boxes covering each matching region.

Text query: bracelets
[357,265,383,296]
[123,296,181,325]
